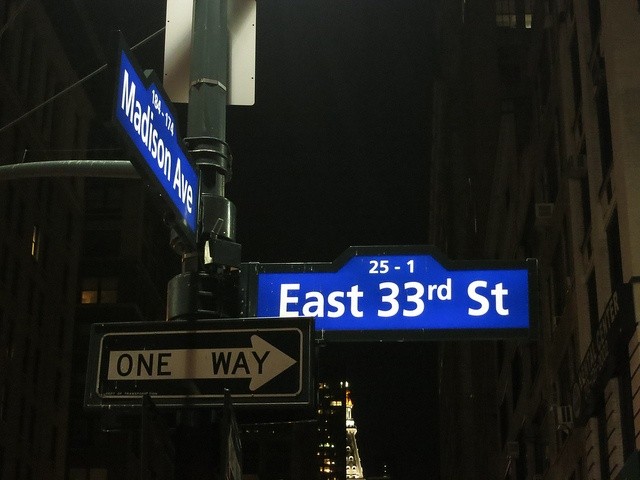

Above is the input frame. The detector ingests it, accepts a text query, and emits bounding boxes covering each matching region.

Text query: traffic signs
[163,0,257,107]
[84,317,312,406]
